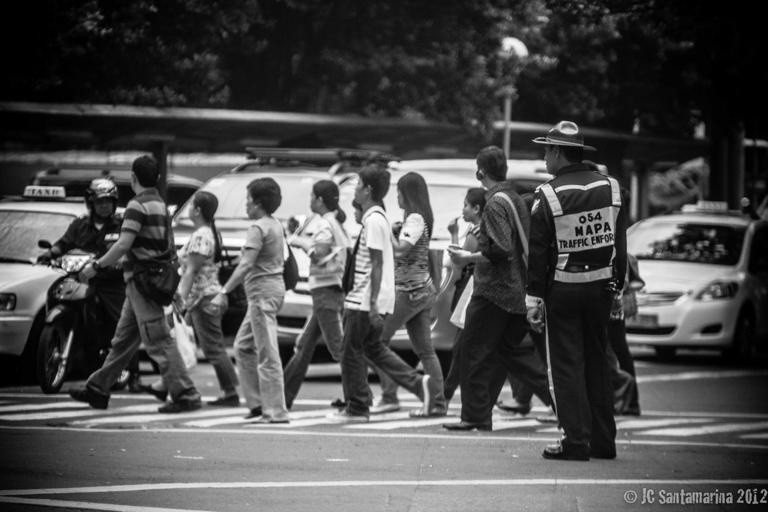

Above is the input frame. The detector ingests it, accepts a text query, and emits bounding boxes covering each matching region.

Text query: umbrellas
[69,384,110,410]
[442,417,494,432]
[138,383,169,401]
[244,406,292,424]
[158,398,203,414]
[207,394,240,407]
[592,441,617,458]
[541,442,590,460]
[496,398,557,422]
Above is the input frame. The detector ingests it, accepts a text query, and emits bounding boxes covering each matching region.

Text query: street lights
[283,253,298,290]
[343,248,356,293]
[500,38,532,158]
[131,255,179,306]
[218,266,249,338]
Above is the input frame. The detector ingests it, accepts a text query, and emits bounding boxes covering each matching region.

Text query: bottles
[531,119,597,151]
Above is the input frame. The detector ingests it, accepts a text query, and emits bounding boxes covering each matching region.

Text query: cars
[1,146,768,396]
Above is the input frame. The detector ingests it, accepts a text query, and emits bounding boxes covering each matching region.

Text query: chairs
[326,410,370,424]
[369,402,402,414]
[409,407,448,417]
[418,373,434,416]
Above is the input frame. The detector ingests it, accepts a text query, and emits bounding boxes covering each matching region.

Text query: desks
[500,38,532,158]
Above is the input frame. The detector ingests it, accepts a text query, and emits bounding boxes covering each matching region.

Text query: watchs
[221,288,230,295]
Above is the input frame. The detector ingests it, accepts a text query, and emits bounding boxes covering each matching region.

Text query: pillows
[85,178,120,204]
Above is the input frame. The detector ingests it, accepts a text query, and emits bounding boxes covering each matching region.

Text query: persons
[283,180,375,410]
[138,189,242,407]
[366,172,447,419]
[439,142,554,432]
[352,198,386,224]
[524,117,630,466]
[207,168,290,425]
[326,170,436,422]
[606,249,646,424]
[36,176,151,396]
[66,153,203,415]
